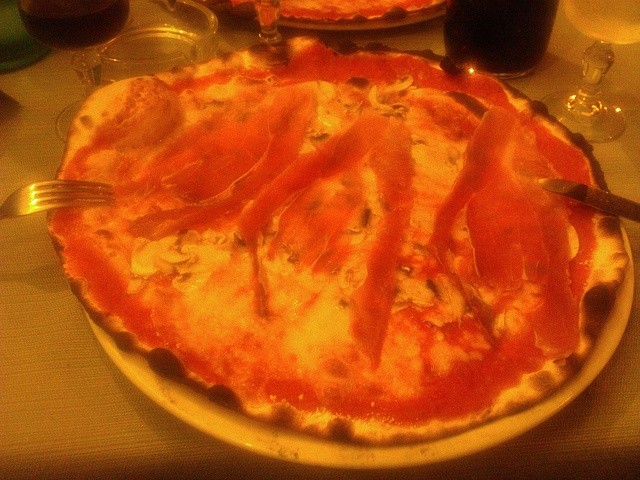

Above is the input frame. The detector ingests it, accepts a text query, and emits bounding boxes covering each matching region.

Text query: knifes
[538,175,640,222]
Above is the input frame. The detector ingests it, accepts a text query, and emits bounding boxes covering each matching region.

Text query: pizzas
[45,33,636,471]
[222,0,452,33]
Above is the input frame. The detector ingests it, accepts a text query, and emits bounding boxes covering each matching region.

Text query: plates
[81,222,636,472]
[279,9,448,35]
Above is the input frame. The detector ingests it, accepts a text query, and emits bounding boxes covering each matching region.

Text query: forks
[1,179,116,221]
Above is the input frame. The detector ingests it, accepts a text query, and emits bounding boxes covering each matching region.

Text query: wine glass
[16,0,132,144]
[249,0,297,68]
[542,0,639,145]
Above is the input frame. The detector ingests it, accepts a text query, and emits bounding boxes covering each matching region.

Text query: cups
[443,0,558,79]
